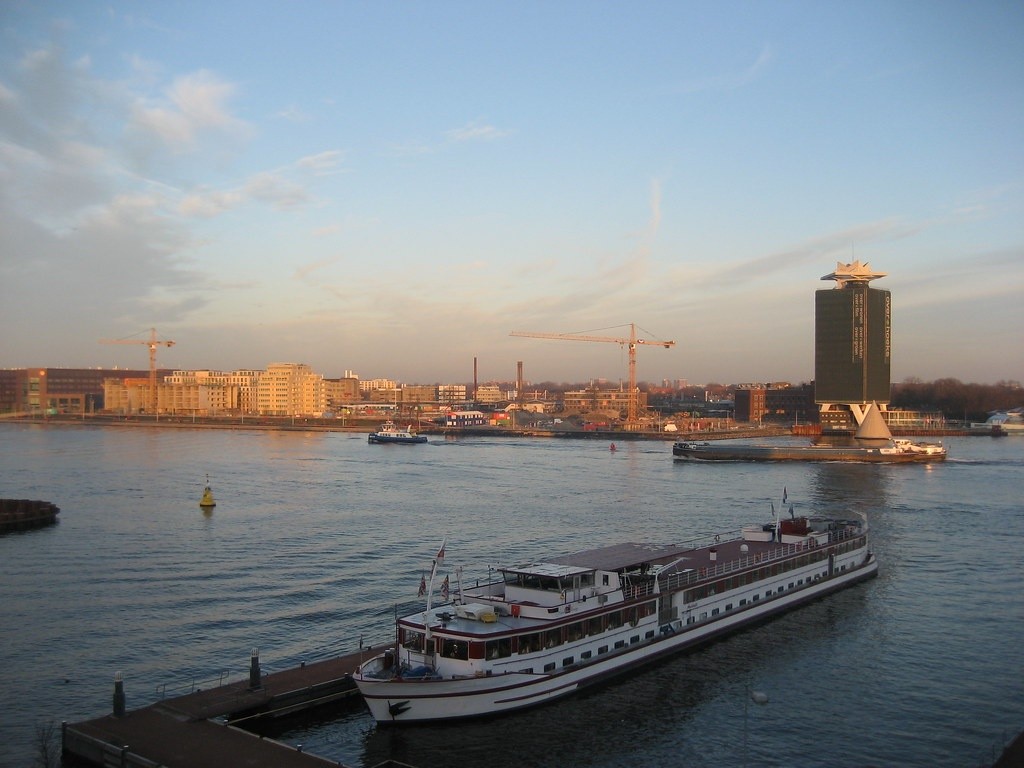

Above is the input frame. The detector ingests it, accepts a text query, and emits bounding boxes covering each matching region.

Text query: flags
[418,572,426,597]
[789,504,794,519]
[783,485,787,503]
[435,539,445,566]
[441,575,450,603]
[771,503,775,517]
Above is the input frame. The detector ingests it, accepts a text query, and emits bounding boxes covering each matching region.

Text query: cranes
[98,327,176,414]
[509,322,676,421]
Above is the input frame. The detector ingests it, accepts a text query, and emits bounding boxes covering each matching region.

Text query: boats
[673,439,950,462]
[368,416,427,443]
[350,485,878,728]
